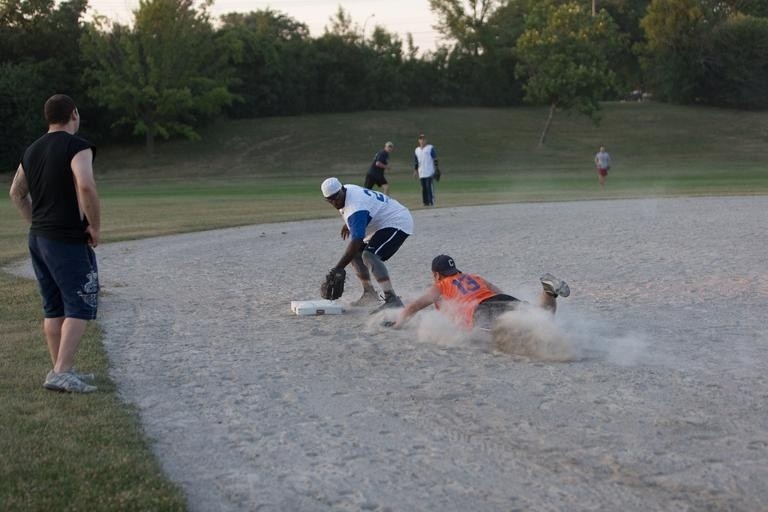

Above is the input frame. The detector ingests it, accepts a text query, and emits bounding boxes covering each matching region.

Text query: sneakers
[352,290,382,306]
[371,295,405,315]
[539,273,570,298]
[42,369,99,393]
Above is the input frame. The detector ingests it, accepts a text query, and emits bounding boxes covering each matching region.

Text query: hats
[432,254,462,276]
[321,177,343,198]
[386,141,393,148]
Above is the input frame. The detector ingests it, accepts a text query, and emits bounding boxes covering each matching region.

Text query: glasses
[419,136,425,140]
[321,193,338,202]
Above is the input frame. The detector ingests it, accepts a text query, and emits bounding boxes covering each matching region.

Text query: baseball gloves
[434,169,441,181]
[320,269,346,302]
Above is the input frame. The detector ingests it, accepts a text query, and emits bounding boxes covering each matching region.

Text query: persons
[9,95,103,393]
[412,134,440,207]
[363,142,394,195]
[394,254,572,340]
[321,176,414,312]
[595,145,611,185]
